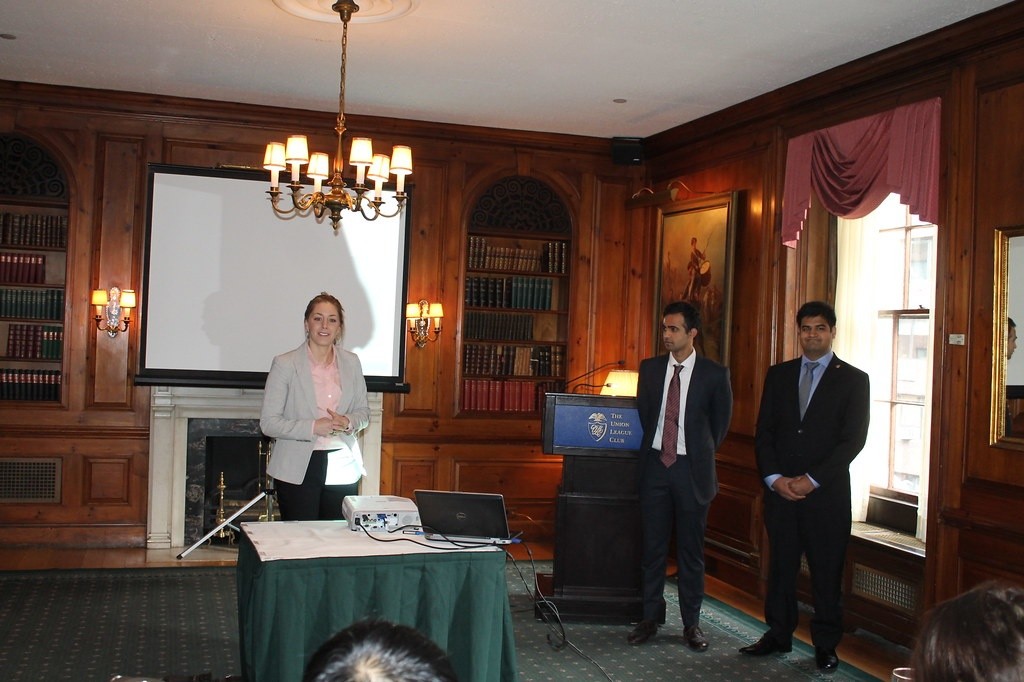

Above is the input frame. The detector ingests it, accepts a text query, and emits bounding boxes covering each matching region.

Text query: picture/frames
[651,190,739,366]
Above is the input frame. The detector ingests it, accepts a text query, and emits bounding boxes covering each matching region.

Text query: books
[0,369,61,401]
[464,380,559,415]
[465,276,552,310]
[0,214,66,247]
[0,290,63,320]
[468,237,565,274]
[0,253,46,284]
[464,345,562,377]
[464,311,533,341]
[7,324,62,359]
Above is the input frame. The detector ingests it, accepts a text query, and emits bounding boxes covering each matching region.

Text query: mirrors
[988,225,1024,452]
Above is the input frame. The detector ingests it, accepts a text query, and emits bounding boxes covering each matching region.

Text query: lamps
[600,370,639,397]
[609,136,645,167]
[92,287,137,339]
[262,0,412,230]
[406,299,444,349]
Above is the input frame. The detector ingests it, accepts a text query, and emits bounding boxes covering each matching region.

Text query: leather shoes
[683,626,708,651]
[739,636,792,655]
[816,646,839,669]
[628,621,653,645]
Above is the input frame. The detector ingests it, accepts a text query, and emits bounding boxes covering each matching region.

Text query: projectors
[342,494,424,532]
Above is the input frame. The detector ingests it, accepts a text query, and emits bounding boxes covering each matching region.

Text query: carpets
[0,559,884,682]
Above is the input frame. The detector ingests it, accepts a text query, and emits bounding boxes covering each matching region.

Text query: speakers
[612,142,643,165]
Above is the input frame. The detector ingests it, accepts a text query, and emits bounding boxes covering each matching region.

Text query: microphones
[559,361,626,393]
[572,383,612,393]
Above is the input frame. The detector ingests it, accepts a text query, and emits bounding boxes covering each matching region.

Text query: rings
[332,430,336,434]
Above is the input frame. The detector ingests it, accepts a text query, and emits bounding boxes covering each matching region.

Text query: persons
[629,302,733,652]
[303,619,457,682]
[1007,317,1017,360]
[739,301,870,671]
[260,295,370,521]
[909,583,1024,682]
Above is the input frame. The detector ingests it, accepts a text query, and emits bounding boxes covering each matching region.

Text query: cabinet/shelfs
[0,244,66,405]
[459,269,571,415]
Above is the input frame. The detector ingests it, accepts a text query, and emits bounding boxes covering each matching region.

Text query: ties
[799,362,820,421]
[660,366,684,468]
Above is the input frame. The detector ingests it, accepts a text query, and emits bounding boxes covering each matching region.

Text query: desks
[237,520,519,682]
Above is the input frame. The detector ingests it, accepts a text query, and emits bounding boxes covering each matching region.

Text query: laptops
[414,489,525,544]
